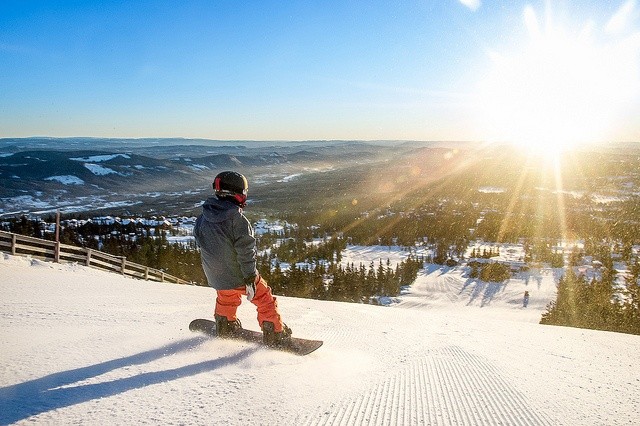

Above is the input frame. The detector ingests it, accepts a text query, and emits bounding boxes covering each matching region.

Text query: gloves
[246,281,257,300]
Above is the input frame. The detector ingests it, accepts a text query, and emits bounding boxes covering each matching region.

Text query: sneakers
[263,322,292,344]
[215,318,241,338]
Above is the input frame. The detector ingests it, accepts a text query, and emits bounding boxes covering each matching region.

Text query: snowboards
[189,319,323,356]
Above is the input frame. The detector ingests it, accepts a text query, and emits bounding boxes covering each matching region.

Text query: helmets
[213,170,248,207]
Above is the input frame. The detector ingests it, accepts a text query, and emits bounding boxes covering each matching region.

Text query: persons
[193,171,292,342]
